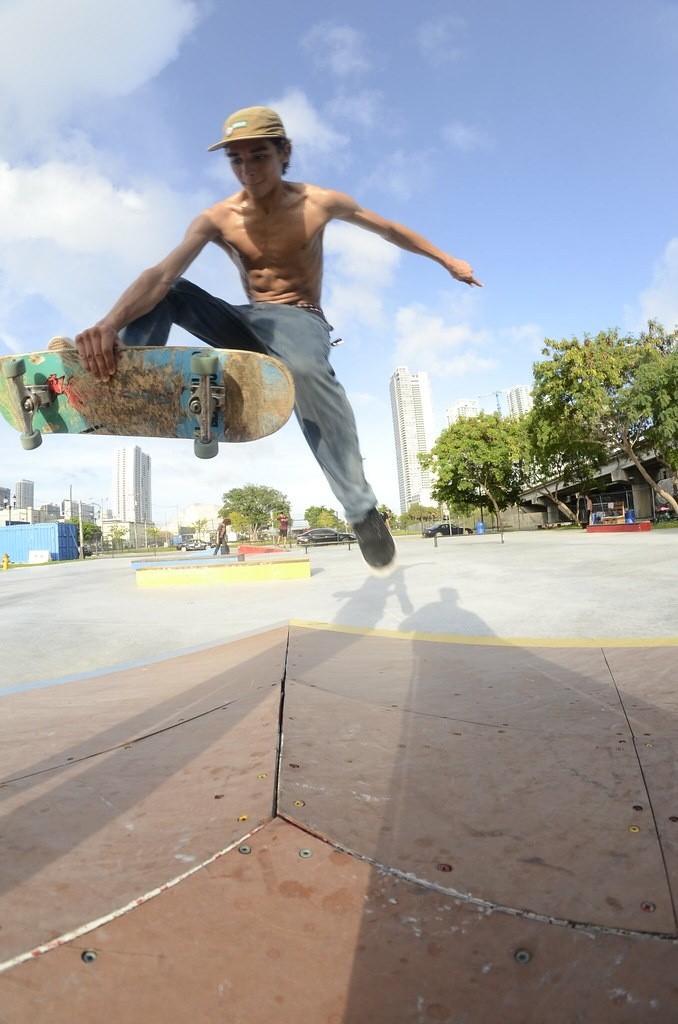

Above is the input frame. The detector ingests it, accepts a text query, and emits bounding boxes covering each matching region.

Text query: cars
[422,523,473,539]
[176,538,219,552]
[78,547,94,557]
[297,527,357,547]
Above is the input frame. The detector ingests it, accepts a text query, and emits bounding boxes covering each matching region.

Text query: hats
[208,107,290,151]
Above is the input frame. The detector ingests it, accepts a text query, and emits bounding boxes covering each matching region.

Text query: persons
[47,108,484,570]
[380,509,391,528]
[213,519,232,555]
[274,511,290,547]
[585,494,593,524]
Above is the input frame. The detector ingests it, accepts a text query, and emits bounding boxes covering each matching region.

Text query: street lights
[2,494,17,525]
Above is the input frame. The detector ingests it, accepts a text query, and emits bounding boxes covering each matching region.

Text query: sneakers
[353,506,399,578]
[48,336,76,352]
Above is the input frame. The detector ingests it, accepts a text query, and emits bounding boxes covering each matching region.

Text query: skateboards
[0,346,296,459]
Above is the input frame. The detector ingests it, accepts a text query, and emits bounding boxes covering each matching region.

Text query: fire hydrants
[2,552,11,570]
